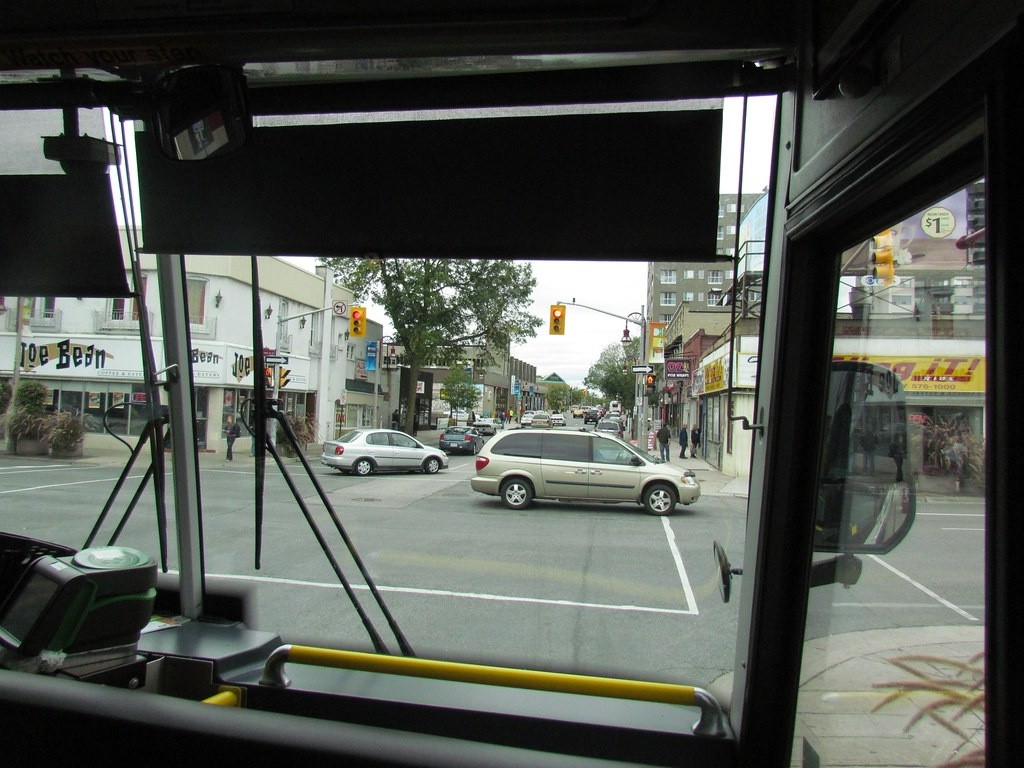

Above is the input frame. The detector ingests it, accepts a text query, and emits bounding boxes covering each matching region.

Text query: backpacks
[233,423,241,438]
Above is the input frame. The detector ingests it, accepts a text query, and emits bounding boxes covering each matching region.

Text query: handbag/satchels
[690,446,696,454]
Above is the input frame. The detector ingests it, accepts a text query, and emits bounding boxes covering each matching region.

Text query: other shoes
[691,455,696,458]
[683,457,687,459]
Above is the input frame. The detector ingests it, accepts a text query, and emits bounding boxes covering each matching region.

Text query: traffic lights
[646,375,656,386]
[550,305,566,335]
[350,307,366,338]
[265,367,275,388]
[871,228,894,286]
[279,368,291,389]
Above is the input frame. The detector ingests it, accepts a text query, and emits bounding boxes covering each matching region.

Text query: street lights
[621,312,648,454]
[623,356,638,406]
[372,336,398,428]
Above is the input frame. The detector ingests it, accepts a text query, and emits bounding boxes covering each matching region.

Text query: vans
[470,428,700,516]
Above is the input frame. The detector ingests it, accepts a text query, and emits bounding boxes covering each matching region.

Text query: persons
[860,425,880,475]
[679,427,688,459]
[392,409,399,430]
[413,412,418,436]
[472,412,475,422]
[499,409,513,424]
[893,434,904,483]
[222,416,236,461]
[657,423,671,462]
[690,424,700,458]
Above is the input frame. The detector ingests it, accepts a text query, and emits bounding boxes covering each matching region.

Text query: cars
[581,406,606,424]
[879,422,907,441]
[595,410,625,439]
[473,419,496,435]
[521,411,553,428]
[321,429,449,477]
[439,426,484,455]
[550,414,567,426]
[448,410,480,421]
[482,418,503,429]
[571,405,584,419]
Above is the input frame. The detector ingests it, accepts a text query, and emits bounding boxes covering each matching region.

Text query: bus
[609,401,622,413]
